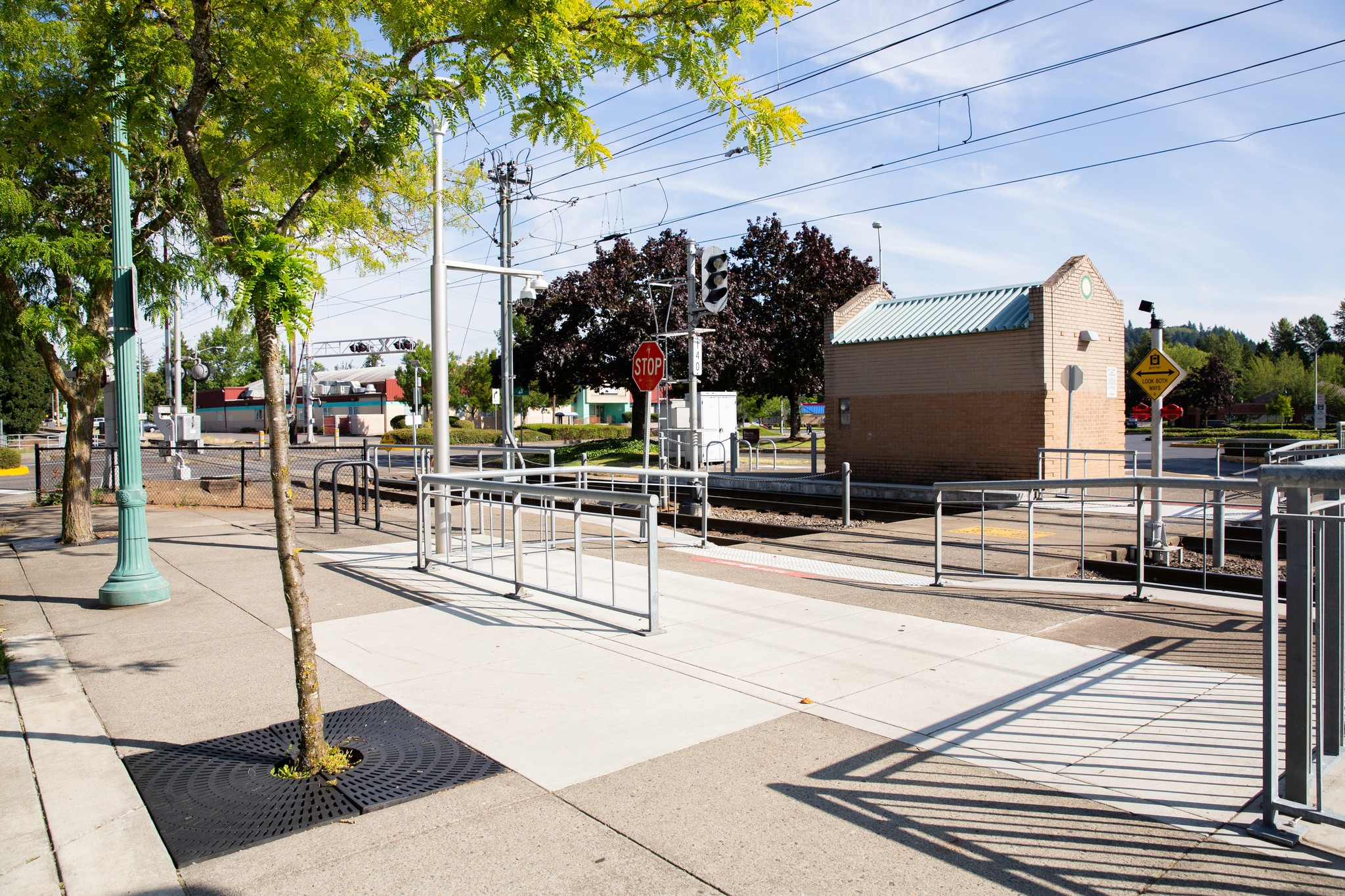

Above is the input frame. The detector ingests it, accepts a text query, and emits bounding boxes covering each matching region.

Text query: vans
[93,418,105,427]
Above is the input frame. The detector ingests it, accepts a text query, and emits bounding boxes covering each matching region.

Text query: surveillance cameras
[519,278,537,308]
[530,275,549,294]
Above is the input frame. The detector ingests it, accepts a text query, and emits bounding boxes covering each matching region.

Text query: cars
[139,422,159,432]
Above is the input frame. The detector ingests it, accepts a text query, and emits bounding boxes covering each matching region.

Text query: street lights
[409,359,428,428]
[192,343,223,413]
[1296,339,1336,429]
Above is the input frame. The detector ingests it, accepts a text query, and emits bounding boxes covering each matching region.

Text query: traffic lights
[350,342,374,352]
[394,339,417,351]
[701,246,730,314]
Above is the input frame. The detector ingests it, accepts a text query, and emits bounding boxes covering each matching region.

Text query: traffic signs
[1135,348,1178,399]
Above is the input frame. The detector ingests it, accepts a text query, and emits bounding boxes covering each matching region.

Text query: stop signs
[632,341,665,392]
